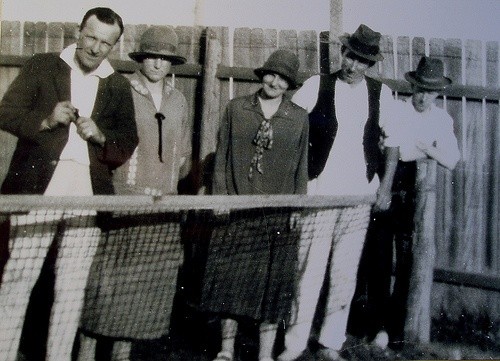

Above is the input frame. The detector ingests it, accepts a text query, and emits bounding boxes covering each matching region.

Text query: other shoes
[213,354,233,361]
[318,348,345,361]
[278,350,307,361]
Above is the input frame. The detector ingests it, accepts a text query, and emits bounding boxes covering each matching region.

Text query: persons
[371,56,460,351]
[0,7,140,360]
[278,22,404,361]
[199,49,310,361]
[78,25,193,361]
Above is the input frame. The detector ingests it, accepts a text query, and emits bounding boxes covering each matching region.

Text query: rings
[88,131,93,138]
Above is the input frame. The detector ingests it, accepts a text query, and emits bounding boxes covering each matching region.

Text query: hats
[128,25,187,65]
[404,55,453,90]
[338,23,385,62]
[253,50,302,91]
